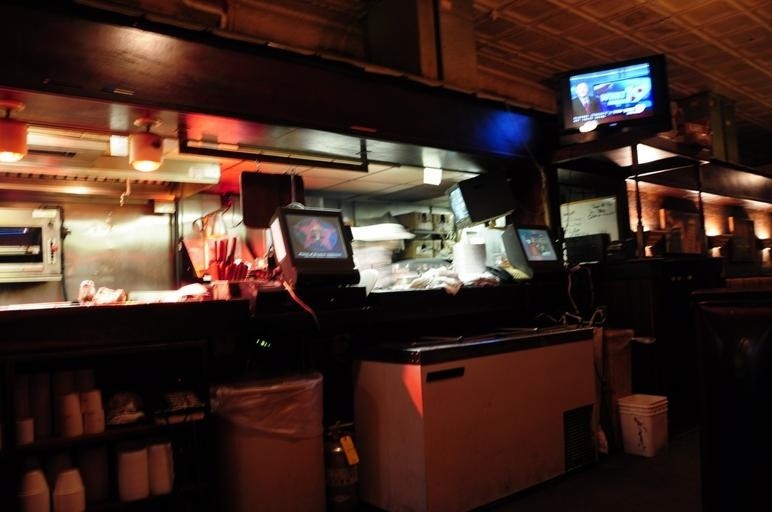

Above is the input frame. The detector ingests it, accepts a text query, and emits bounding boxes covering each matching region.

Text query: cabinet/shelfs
[0,298,254,512]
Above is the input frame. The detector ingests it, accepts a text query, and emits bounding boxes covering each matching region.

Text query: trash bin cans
[214,371,326,511]
[600,328,667,458]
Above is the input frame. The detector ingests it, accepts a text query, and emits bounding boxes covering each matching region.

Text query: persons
[571,82,603,118]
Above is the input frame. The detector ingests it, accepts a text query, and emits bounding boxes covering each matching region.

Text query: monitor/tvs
[501,221,561,277]
[238,170,307,228]
[275,207,355,289]
[444,172,516,227]
[559,55,670,132]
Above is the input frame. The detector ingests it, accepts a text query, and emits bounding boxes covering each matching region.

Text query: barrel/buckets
[615,393,668,458]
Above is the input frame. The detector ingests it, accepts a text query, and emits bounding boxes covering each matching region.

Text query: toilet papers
[148,442,174,495]
[16,418,34,444]
[62,389,108,439]
[115,450,148,501]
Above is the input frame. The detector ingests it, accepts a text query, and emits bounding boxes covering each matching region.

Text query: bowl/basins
[19,391,175,509]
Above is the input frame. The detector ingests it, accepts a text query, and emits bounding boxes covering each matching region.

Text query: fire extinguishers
[328,421,358,504]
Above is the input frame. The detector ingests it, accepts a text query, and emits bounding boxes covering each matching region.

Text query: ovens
[1,201,62,289]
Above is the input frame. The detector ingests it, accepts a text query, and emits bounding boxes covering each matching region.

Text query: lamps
[0,98,29,164]
[127,118,164,172]
